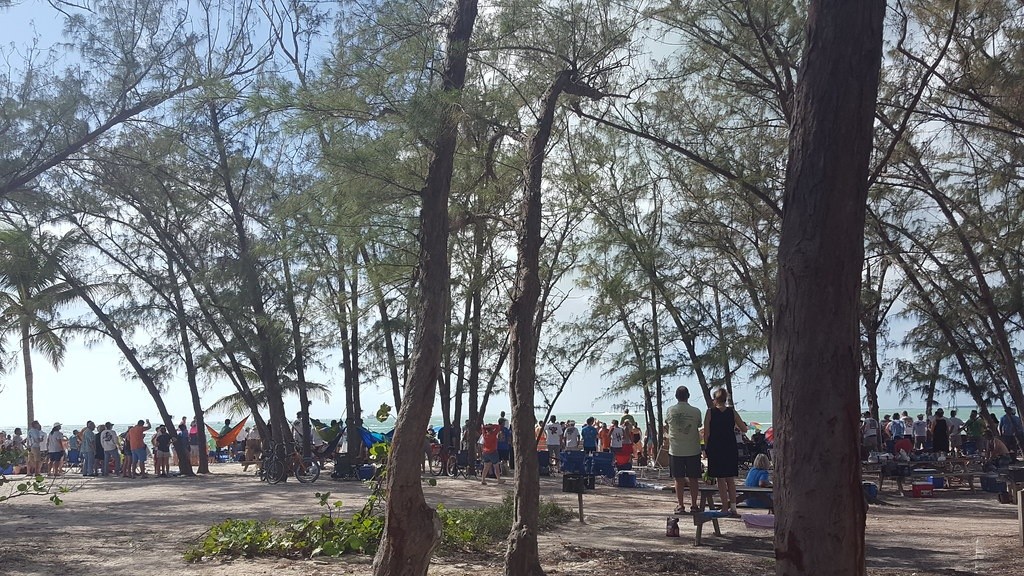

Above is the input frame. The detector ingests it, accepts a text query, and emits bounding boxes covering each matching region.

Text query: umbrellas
[748,422,763,430]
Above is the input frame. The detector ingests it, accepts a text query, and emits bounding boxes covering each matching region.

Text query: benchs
[864,470,914,474]
[690,510,776,546]
[241,461,263,472]
[699,487,773,513]
[911,471,999,478]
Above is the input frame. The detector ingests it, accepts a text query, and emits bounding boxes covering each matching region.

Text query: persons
[0,411,367,479]
[665,386,702,514]
[427,409,657,484]
[745,453,771,508]
[751,429,765,441]
[703,388,747,514]
[861,407,1024,463]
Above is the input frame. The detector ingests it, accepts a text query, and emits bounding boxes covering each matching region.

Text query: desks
[877,457,978,498]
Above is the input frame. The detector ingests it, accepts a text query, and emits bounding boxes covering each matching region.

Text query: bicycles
[430,444,458,477]
[258,441,321,484]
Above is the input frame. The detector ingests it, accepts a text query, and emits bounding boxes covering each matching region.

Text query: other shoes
[498,480,505,484]
[729,510,741,518]
[482,482,487,485]
[689,506,702,513]
[674,505,685,513]
[25,466,172,479]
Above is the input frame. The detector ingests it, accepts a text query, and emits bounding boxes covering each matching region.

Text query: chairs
[330,452,358,481]
[560,449,616,487]
[737,442,770,471]
[536,450,556,478]
[632,444,670,481]
[64,449,83,474]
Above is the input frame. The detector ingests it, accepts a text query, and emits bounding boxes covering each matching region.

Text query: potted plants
[702,469,717,485]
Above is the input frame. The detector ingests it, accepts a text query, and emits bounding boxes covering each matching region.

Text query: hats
[569,420,577,425]
[1012,407,1016,414]
[498,418,506,422]
[245,427,251,431]
[105,422,114,427]
[54,422,62,427]
[862,411,870,415]
